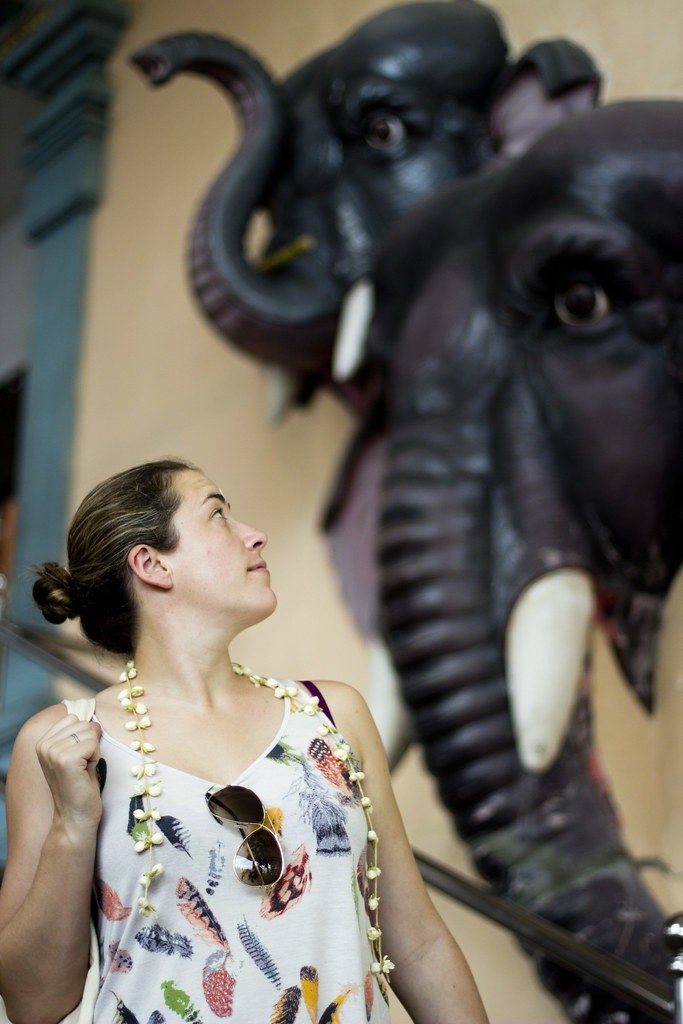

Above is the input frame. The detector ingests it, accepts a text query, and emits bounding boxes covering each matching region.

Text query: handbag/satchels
[0,916,100,1024]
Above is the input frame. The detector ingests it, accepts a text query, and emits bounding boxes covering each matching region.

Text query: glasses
[203,784,283,891]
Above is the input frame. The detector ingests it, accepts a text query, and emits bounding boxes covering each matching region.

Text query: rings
[71,734,80,743]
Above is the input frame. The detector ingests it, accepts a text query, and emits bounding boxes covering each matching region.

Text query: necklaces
[120,659,395,979]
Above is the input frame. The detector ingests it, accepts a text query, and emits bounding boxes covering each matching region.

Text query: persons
[0,462,492,1024]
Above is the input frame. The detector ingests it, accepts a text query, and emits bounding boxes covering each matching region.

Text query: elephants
[378,99,683,1024]
[129,0,603,440]
